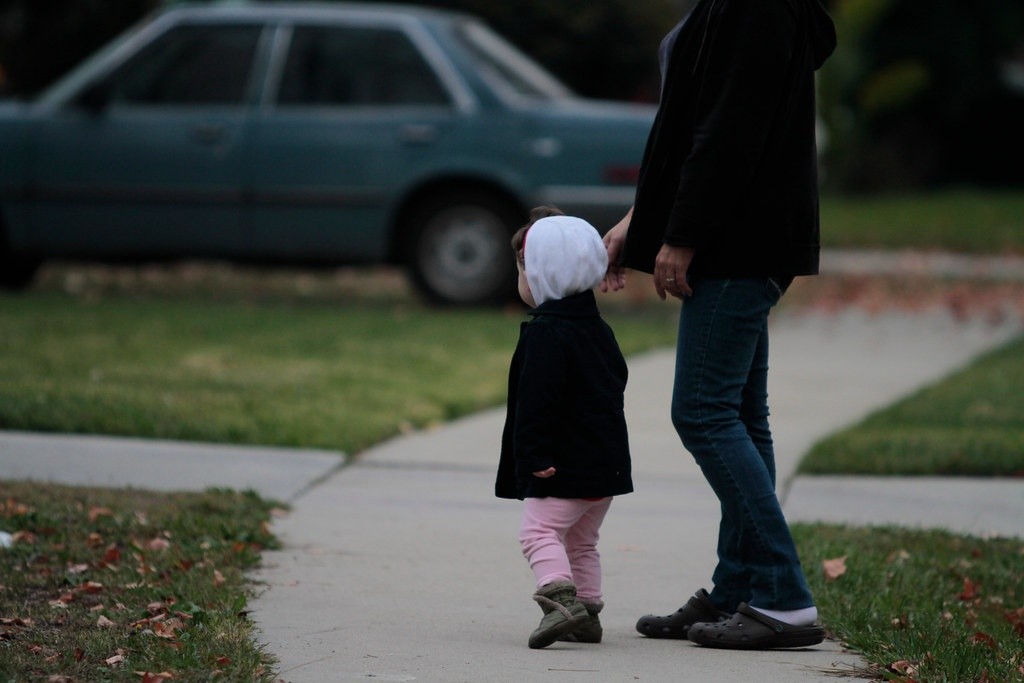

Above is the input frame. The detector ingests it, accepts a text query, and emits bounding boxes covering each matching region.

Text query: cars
[1,1,661,308]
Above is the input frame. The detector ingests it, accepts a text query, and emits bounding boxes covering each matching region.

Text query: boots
[558,601,604,643]
[528,578,588,648]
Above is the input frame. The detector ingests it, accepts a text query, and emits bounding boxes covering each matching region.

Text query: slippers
[636,588,733,638]
[688,601,825,650]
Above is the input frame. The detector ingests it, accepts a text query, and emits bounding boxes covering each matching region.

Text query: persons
[493,209,635,648]
[603,0,838,651]
